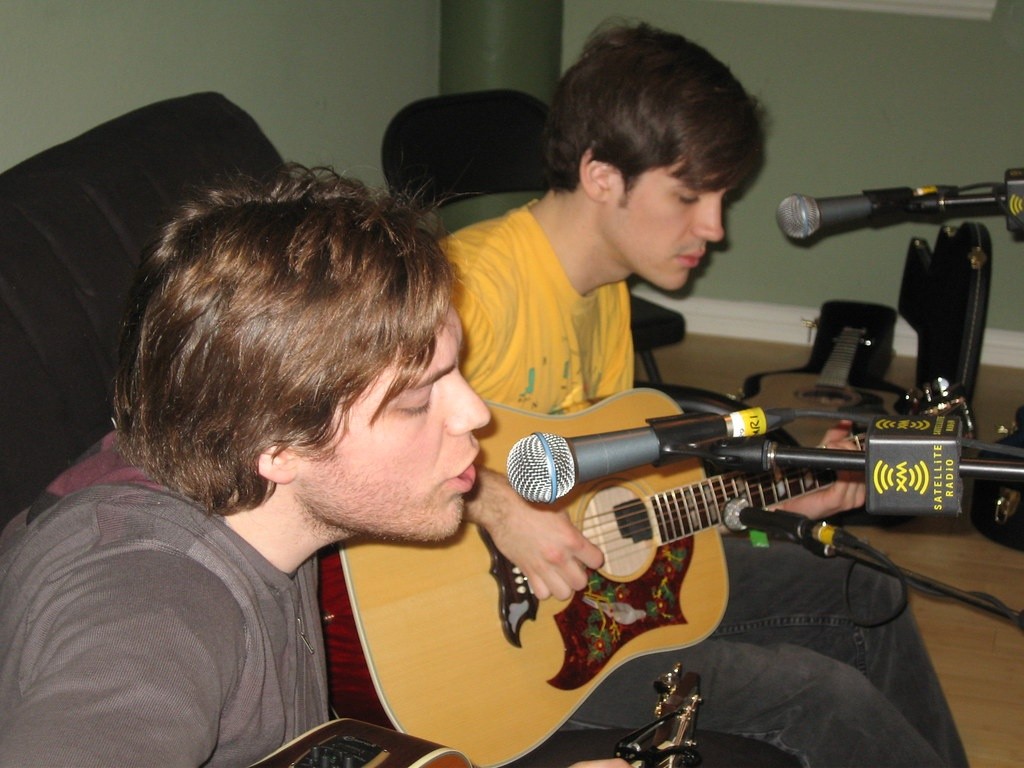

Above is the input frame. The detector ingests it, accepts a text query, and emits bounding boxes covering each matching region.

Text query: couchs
[1,92,803,768]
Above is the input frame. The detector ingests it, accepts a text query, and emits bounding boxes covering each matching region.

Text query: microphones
[722,497,860,549]
[776,185,959,240]
[507,408,799,503]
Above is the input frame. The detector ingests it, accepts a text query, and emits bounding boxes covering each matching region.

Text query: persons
[0,164,638,768]
[435,16,967,768]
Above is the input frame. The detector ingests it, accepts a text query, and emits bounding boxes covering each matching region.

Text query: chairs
[380,89,685,383]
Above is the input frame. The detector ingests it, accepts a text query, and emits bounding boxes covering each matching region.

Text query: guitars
[309,377,978,768]
[243,661,704,768]
[737,322,902,478]
